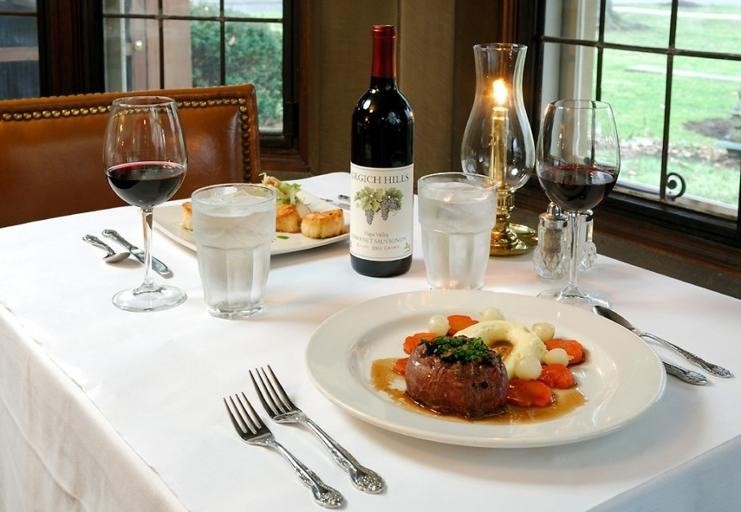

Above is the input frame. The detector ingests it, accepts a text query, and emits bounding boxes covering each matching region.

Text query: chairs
[0,84,260,231]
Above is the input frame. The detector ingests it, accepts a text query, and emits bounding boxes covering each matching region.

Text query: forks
[224,363,386,509]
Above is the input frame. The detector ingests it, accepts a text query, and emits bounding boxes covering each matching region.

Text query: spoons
[83,233,131,262]
[593,304,735,381]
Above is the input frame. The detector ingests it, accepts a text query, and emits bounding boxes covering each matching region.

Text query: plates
[304,289,667,449]
[153,190,356,260]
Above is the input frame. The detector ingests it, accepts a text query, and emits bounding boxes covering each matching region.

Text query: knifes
[103,228,173,278]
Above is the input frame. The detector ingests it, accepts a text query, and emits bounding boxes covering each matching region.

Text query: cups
[192,183,277,319]
[417,171,500,288]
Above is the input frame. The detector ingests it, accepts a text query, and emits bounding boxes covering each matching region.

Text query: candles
[488,107,508,193]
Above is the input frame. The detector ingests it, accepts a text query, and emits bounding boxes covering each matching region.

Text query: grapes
[364,196,400,226]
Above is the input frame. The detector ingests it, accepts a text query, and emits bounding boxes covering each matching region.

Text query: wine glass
[536,97,620,311]
[103,96,188,313]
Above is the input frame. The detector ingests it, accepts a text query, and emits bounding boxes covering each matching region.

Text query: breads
[300,208,344,239]
[182,201,192,231]
[276,204,301,234]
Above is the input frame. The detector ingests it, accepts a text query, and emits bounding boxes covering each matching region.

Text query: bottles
[539,201,570,278]
[350,23,414,278]
